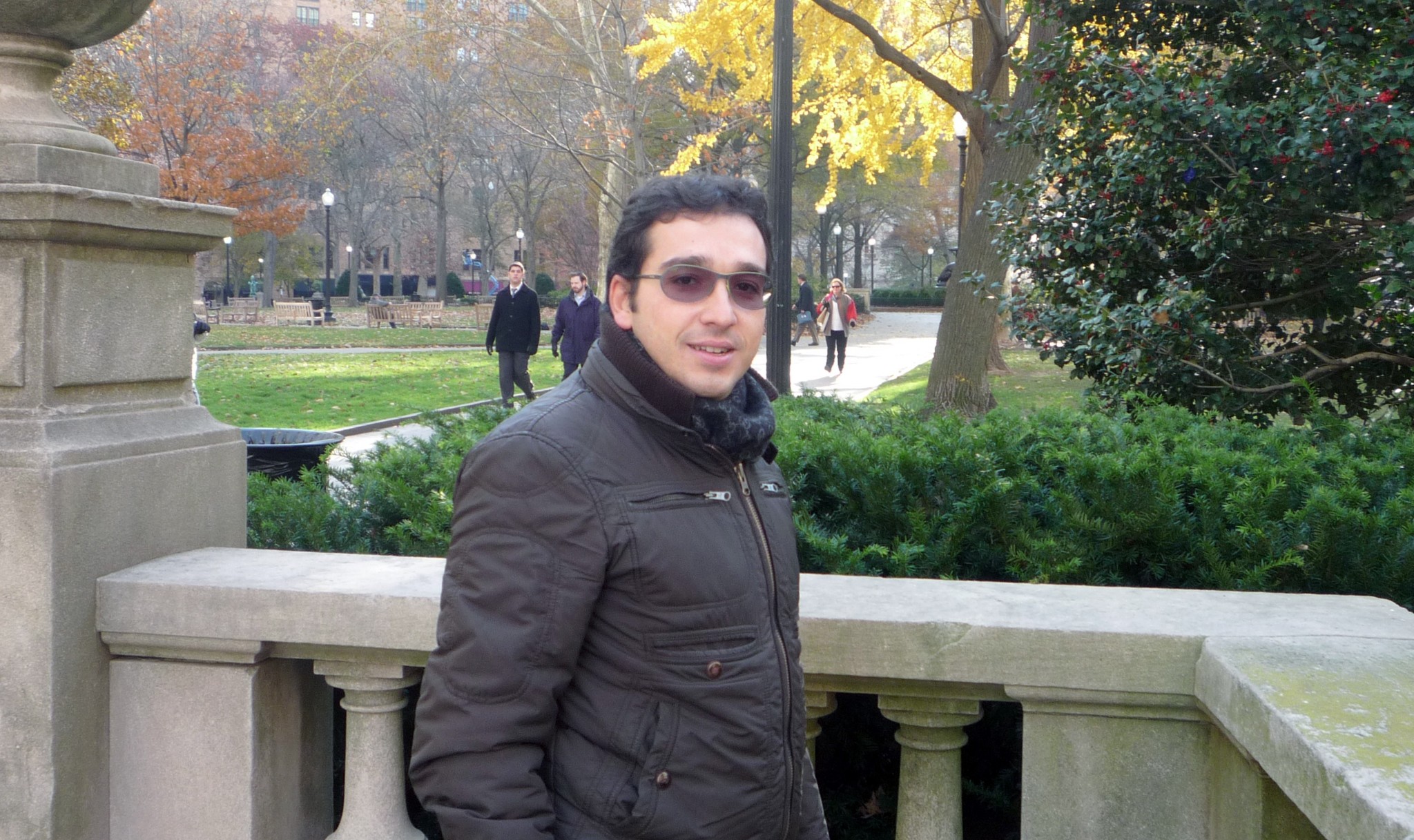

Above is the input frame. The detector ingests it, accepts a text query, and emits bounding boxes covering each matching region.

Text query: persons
[370,293,396,328]
[551,271,602,382]
[790,274,820,346]
[408,171,832,840]
[816,278,857,373]
[486,262,541,409]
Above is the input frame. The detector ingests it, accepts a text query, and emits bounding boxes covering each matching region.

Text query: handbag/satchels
[817,306,830,332]
[796,310,813,326]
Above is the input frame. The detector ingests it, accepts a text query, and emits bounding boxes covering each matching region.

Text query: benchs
[192,297,493,331]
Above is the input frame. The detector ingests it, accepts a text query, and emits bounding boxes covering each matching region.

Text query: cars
[203,280,250,303]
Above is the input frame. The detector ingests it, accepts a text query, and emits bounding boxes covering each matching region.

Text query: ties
[511,288,517,298]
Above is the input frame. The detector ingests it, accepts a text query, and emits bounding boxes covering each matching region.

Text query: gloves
[487,346,494,355]
[552,345,559,357]
[850,321,855,327]
[527,345,538,355]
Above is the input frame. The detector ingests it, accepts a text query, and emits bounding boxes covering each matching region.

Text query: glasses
[832,286,840,288]
[633,264,775,311]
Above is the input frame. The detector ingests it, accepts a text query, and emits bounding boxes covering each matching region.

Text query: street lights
[470,252,477,296]
[868,238,876,293]
[258,258,263,278]
[951,111,967,246]
[843,273,848,289]
[321,188,337,322]
[346,244,352,269]
[817,205,826,277]
[223,236,231,306]
[927,247,933,287]
[833,223,841,279]
[516,228,524,263]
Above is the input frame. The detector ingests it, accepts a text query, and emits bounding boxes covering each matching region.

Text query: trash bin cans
[242,426,346,491]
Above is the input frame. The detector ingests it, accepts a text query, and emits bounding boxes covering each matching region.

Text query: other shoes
[791,340,796,346]
[808,342,819,346]
